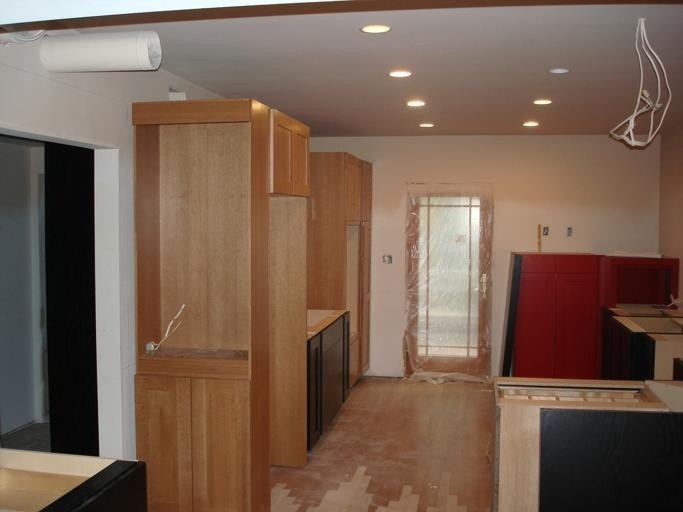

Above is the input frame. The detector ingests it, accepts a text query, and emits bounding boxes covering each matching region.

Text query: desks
[492,376,682,512]
[603,304,683,382]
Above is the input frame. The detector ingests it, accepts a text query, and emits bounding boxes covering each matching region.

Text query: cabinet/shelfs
[308,151,372,390]
[306,307,349,452]
[134,99,310,511]
[0,446,145,512]
